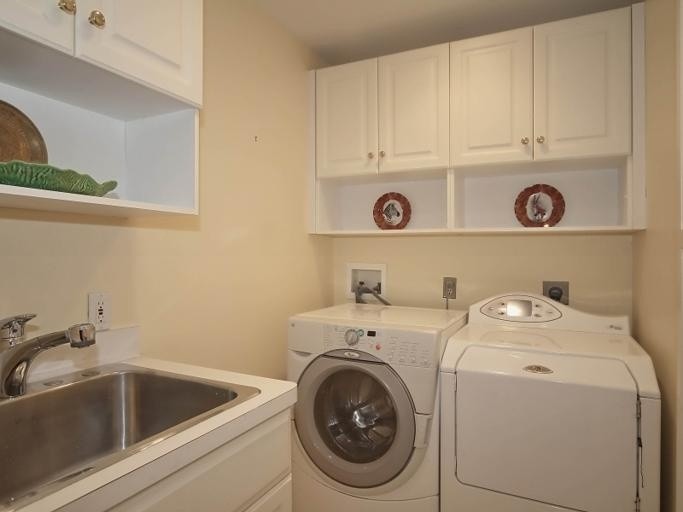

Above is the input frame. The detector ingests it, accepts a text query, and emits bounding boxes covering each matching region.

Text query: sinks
[1,360,261,512]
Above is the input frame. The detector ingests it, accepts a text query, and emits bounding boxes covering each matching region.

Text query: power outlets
[87,293,111,330]
[542,281,569,306]
[442,277,457,298]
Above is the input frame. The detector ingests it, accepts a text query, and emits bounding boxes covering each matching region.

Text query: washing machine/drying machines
[287,302,466,511]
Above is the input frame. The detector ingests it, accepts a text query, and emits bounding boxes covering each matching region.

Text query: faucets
[0,314,95,398]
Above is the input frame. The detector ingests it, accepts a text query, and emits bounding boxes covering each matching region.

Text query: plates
[373,192,412,230]
[0,160,117,197]
[513,183,566,228]
[0,97,49,166]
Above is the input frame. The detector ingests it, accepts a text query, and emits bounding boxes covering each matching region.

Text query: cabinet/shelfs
[306,42,449,236]
[448,1,632,233]
[53,383,298,511]
[0,1,202,217]
